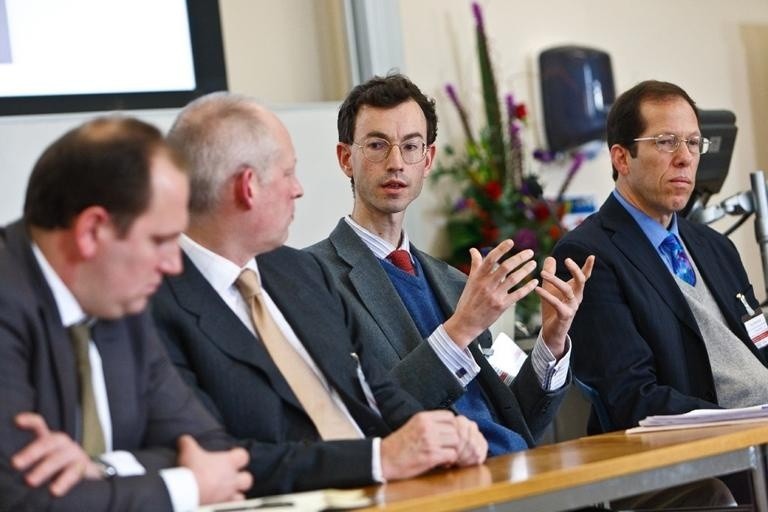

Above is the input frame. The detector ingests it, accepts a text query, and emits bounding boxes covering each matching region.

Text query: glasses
[634,132,712,157]
[350,140,431,164]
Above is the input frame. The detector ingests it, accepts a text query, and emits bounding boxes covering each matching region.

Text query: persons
[549,80,768,435]
[147,89,491,499]
[293,66,741,511]
[0,109,255,511]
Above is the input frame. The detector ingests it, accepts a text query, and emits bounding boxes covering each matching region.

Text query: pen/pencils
[214,502,294,512]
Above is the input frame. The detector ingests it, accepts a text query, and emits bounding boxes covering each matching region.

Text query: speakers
[697,110,738,199]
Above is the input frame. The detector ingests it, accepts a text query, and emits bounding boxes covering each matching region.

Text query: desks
[320,418,768,512]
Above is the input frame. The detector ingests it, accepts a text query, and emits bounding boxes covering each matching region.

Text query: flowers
[421,0,585,335]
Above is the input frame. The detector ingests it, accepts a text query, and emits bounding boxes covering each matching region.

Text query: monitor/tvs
[535,42,617,161]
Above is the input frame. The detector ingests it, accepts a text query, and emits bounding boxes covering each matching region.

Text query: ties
[664,234,697,288]
[68,323,108,459]
[389,250,417,274]
[234,269,363,440]
